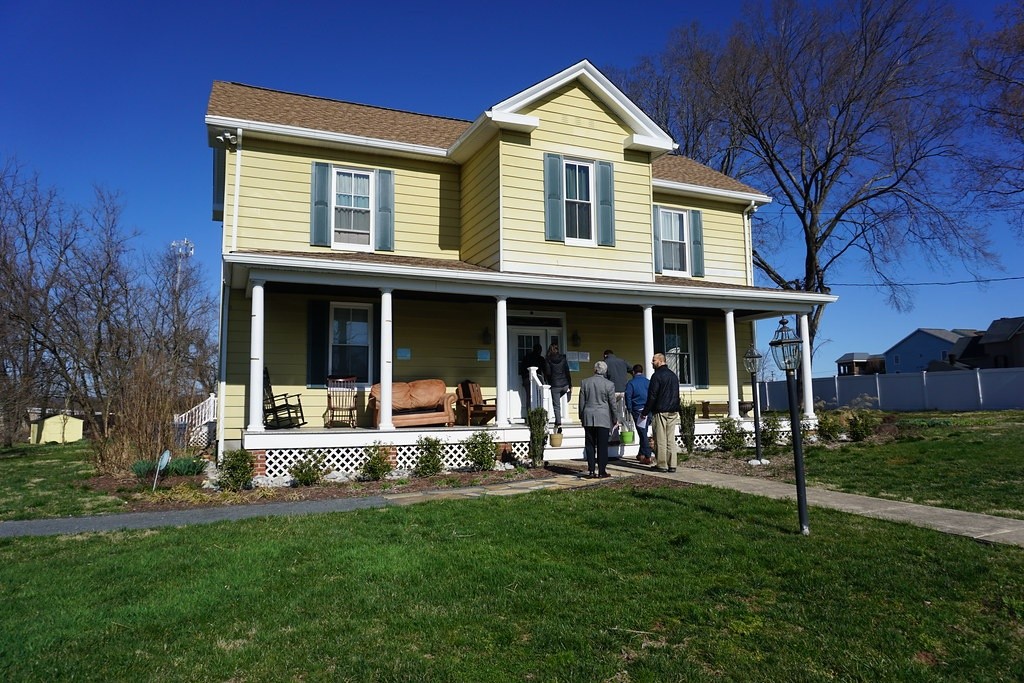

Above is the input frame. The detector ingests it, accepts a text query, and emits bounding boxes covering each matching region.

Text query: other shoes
[639,458,651,464]
[651,465,669,472]
[589,473,594,477]
[598,473,611,477]
[636,455,644,460]
[668,467,676,472]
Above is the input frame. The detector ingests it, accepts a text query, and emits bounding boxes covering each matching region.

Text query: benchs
[691,403,729,419]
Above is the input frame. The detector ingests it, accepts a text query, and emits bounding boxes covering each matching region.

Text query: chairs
[322,376,358,429]
[458,380,497,426]
[262,366,307,430]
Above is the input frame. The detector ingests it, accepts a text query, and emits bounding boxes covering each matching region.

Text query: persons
[543,344,572,433]
[579,361,619,479]
[518,343,545,424]
[602,349,634,446]
[624,364,654,464]
[639,353,681,472]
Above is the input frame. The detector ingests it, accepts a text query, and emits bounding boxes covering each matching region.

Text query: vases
[738,401,752,416]
[551,433,564,447]
[621,432,634,443]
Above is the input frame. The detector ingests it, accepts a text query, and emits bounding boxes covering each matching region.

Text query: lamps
[215,132,236,145]
[749,206,758,213]
[482,329,493,345]
[572,330,581,346]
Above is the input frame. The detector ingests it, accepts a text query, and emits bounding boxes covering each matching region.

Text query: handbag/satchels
[567,391,571,402]
[609,423,620,446]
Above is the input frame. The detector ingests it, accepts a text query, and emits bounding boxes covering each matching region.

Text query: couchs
[368,378,458,429]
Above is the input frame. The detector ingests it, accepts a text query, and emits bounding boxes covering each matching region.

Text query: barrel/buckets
[621,431,633,444]
[550,433,563,448]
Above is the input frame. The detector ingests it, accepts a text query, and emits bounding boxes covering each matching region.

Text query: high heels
[557,427,562,434]
